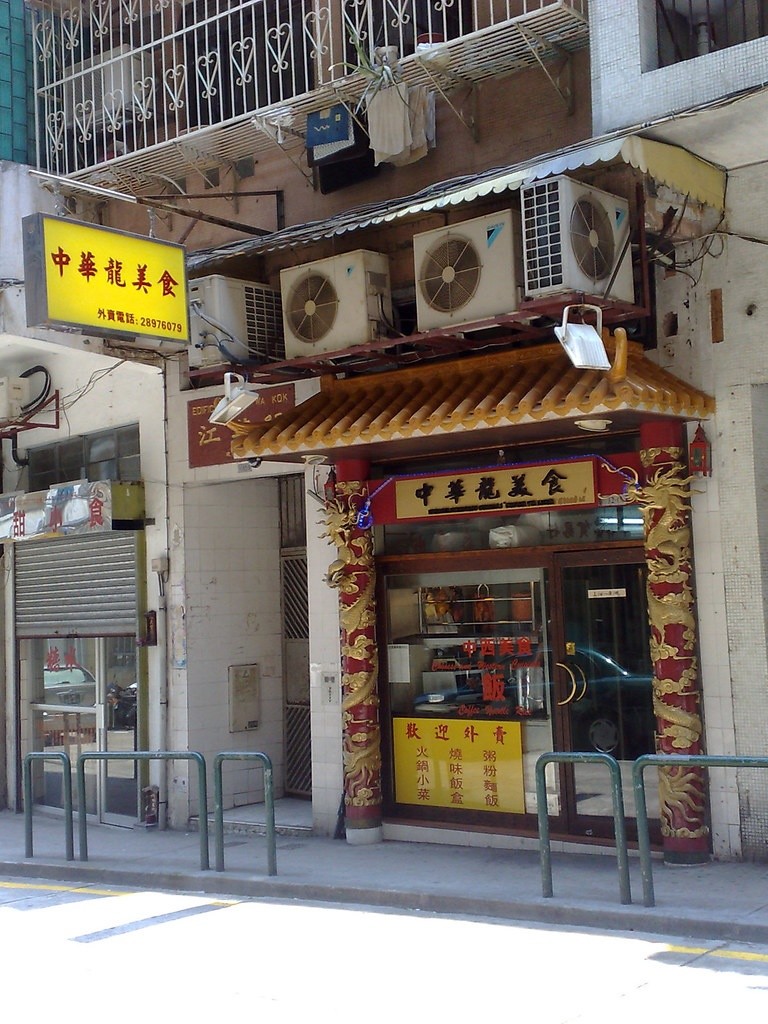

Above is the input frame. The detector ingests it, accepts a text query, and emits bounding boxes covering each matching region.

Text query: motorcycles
[107,673,137,730]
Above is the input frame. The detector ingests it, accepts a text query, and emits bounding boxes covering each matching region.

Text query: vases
[374,45,399,64]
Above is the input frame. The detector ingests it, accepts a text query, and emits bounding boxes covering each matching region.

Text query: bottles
[42,727,95,746]
[516,662,544,713]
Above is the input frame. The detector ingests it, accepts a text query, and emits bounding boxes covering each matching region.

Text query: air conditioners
[519,174,636,305]
[413,208,534,333]
[279,248,392,362]
[174,273,285,370]
[59,44,155,132]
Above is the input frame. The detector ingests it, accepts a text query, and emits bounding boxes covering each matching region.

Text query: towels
[305,101,355,161]
[365,82,437,167]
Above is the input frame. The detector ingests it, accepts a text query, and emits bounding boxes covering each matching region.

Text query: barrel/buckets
[511,592,532,621]
[472,584,496,621]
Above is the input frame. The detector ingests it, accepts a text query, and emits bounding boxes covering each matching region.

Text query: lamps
[207,372,259,429]
[553,300,612,373]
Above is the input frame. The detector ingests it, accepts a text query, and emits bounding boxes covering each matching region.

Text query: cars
[43,664,96,741]
[411,644,653,757]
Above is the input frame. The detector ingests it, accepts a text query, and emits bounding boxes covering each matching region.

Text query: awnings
[183,136,728,273]
